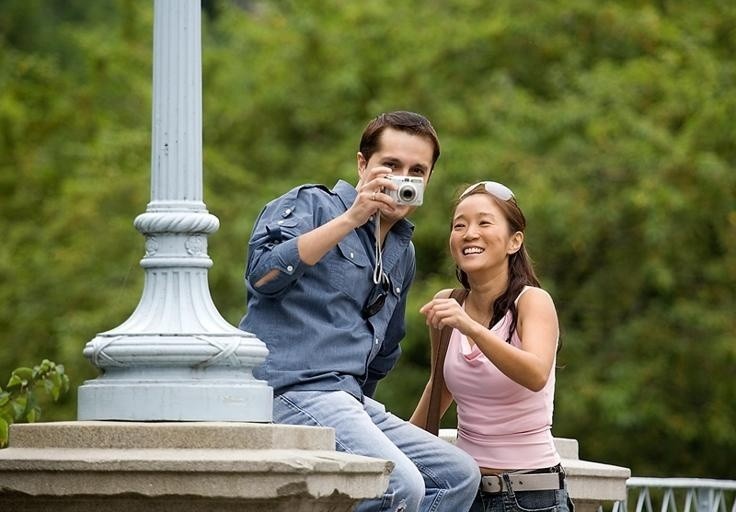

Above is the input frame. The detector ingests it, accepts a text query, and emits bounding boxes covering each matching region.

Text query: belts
[480,470,564,493]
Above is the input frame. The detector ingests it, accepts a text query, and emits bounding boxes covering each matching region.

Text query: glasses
[361,264,390,319]
[457,180,520,204]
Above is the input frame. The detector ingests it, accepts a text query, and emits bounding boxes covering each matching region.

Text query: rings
[372,192,375,201]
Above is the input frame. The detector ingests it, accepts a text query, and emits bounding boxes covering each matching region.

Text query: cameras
[383,175,424,207]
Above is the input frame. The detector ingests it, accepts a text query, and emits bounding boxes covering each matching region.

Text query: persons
[237,109,482,511]
[409,179,577,511]
